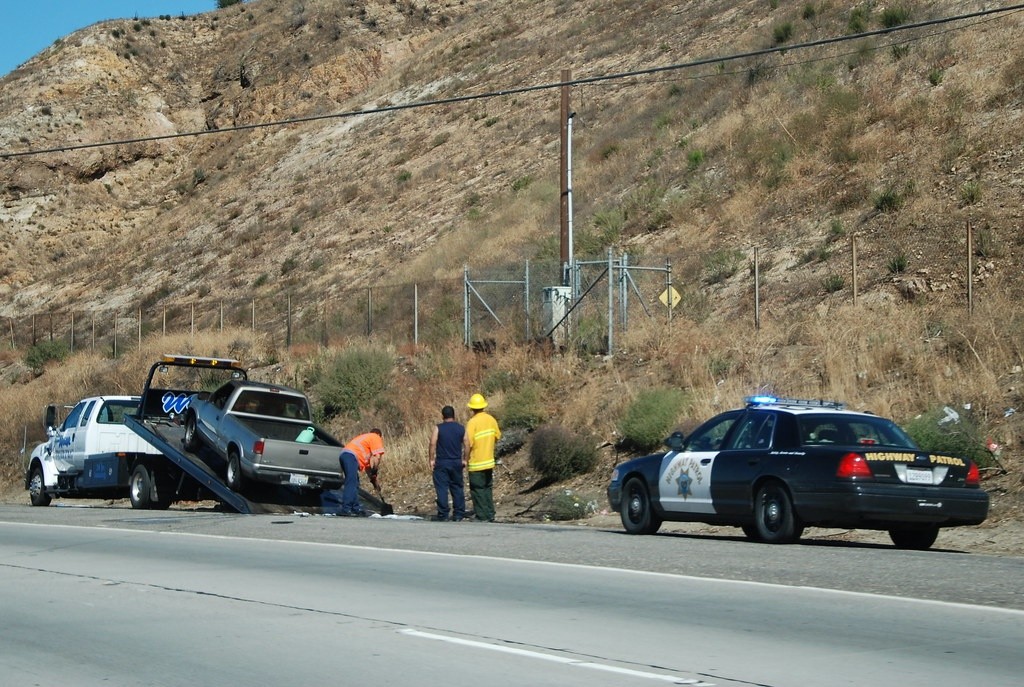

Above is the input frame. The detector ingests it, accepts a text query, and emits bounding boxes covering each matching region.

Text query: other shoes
[453,516,463,522]
[469,515,488,523]
[431,515,448,522]
[352,509,365,515]
[342,511,357,517]
[490,516,495,522]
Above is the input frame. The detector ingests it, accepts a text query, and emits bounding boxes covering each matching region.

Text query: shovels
[371,476,393,516]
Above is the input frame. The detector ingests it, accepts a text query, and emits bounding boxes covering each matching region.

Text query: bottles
[295,426,315,444]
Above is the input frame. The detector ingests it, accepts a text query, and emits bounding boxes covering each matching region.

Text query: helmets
[467,394,488,409]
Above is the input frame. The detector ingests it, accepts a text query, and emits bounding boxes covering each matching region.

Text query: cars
[605,395,991,550]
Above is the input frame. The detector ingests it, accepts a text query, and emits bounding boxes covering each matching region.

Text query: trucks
[23,354,394,515]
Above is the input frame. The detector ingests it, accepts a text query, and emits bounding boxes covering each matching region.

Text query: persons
[429,394,501,523]
[336,428,384,517]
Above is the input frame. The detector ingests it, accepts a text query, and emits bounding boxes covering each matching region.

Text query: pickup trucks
[184,379,352,494]
